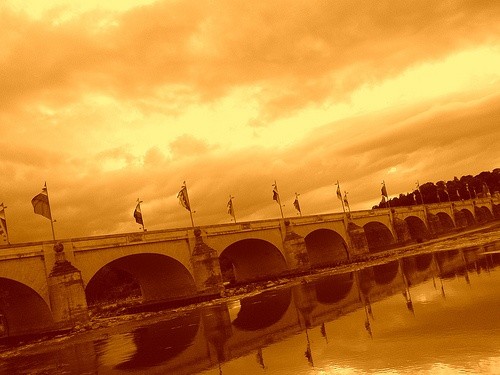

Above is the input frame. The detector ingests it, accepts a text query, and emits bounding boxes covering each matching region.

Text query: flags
[413,181,488,201]
[134,203,144,225]
[335,185,348,206]
[32,186,51,220]
[177,186,190,211]
[382,185,388,197]
[0,204,6,236]
[216,262,471,375]
[228,200,235,217]
[273,186,280,205]
[293,197,300,213]
